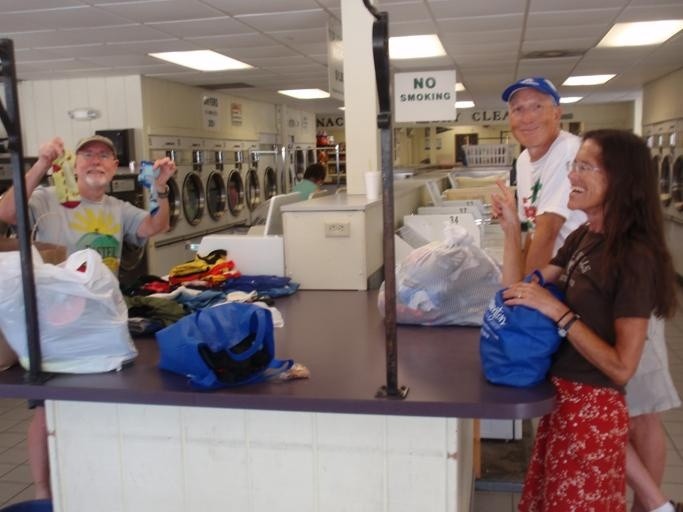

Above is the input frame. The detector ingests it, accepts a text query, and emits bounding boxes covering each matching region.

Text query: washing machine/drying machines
[251,151,282,202]
[639,117,682,226]
[289,143,318,190]
[228,139,245,230]
[207,137,227,232]
[149,134,182,246]
[242,141,262,224]
[182,136,206,239]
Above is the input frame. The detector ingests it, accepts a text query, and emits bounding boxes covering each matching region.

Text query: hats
[502,76,560,104]
[75,135,116,156]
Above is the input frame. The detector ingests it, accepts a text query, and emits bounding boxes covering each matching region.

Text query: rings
[518,291,521,298]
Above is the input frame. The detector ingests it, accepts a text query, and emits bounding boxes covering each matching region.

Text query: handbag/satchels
[0,196,69,376]
[480,269,564,388]
[154,303,294,388]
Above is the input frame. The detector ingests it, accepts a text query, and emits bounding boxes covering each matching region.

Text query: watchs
[157,184,170,198]
[557,313,581,337]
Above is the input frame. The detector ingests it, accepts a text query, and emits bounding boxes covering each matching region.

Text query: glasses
[567,159,603,175]
[78,151,114,159]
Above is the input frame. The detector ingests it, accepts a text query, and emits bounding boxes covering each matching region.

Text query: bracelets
[554,309,571,326]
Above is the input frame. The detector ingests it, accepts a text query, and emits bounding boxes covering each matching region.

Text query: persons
[489,128,678,512]
[292,163,326,201]
[1,133,177,501]
[502,74,682,512]
